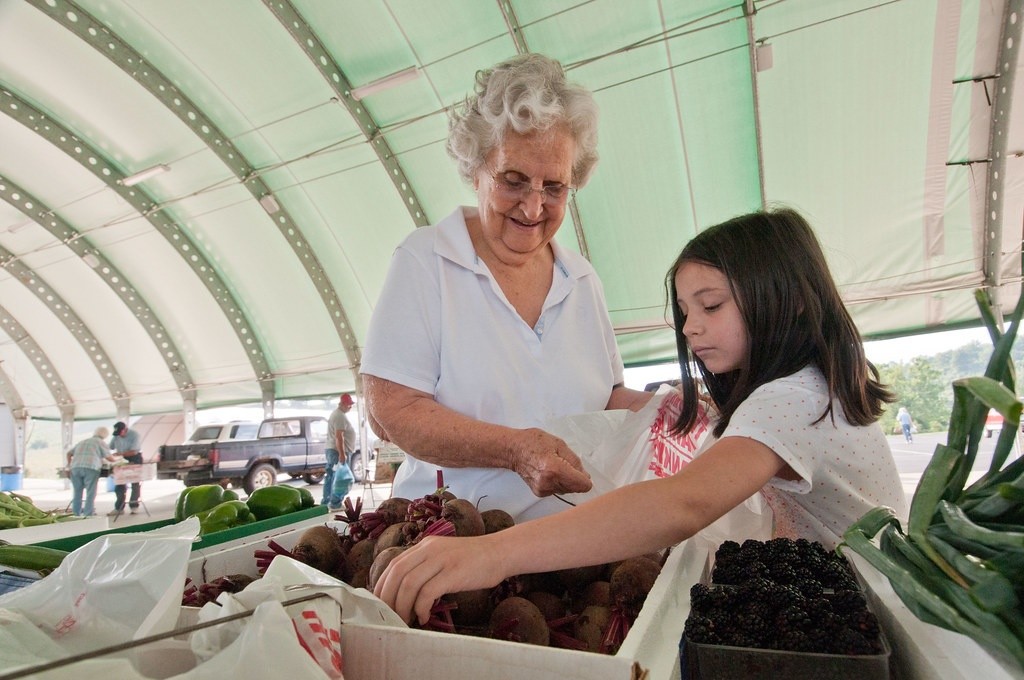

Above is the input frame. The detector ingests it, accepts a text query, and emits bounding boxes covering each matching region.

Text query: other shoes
[329,504,346,512]
[80,508,84,513]
[130,507,140,514]
[107,509,124,515]
[86,512,97,516]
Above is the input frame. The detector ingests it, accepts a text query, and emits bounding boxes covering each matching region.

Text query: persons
[68,428,124,515]
[356,52,720,525]
[108,421,143,514]
[321,394,356,512]
[373,203,908,625]
[896,405,913,442]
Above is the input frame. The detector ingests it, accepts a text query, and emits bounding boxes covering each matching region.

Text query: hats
[341,393,355,405]
[113,422,125,436]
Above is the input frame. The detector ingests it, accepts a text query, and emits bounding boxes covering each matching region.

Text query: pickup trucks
[156,416,373,497]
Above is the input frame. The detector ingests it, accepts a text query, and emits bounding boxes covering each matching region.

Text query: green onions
[844,289,1024,680]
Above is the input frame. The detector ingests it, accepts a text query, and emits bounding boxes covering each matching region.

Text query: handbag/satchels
[331,460,355,499]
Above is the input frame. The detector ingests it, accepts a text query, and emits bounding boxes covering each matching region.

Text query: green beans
[0,490,75,528]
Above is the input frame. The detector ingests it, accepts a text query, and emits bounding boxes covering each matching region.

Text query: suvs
[184,420,259,442]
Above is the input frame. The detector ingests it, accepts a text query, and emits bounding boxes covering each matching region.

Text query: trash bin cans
[0,465,23,491]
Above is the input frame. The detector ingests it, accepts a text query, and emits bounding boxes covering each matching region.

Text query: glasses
[480,154,578,203]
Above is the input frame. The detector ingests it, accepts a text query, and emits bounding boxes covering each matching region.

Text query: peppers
[174,483,314,535]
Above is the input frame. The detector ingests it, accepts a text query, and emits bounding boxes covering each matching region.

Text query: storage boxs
[0,513,1023,680]
[374,445,404,484]
